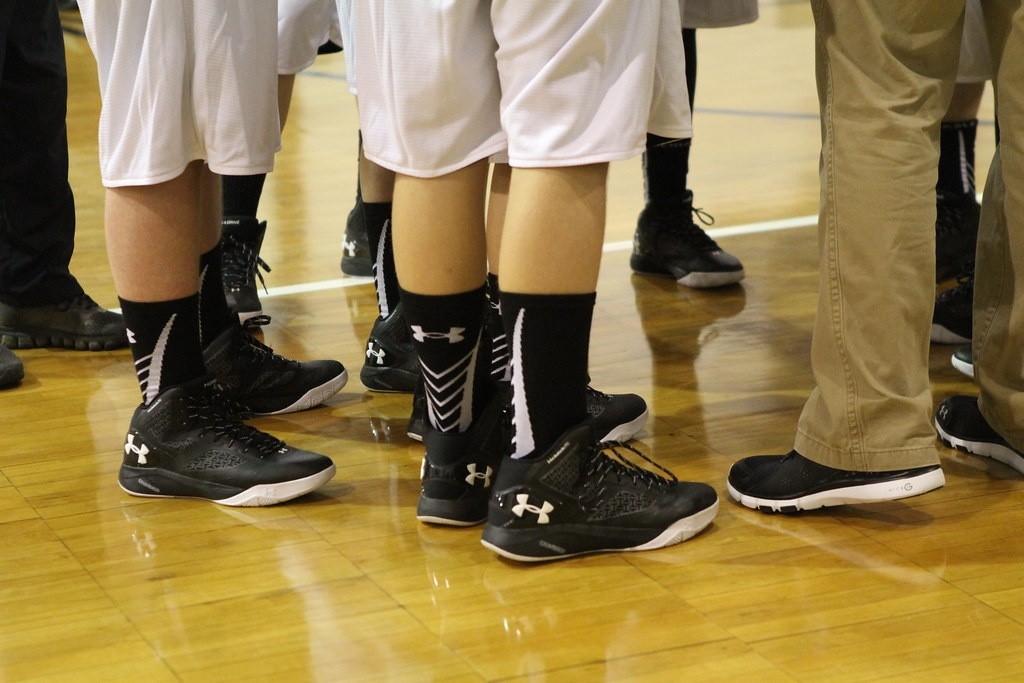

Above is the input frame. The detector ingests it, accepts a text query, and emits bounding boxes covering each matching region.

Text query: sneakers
[194,313,349,415]
[0,340,27,389]
[926,268,975,346]
[477,420,721,565]
[0,293,130,350]
[630,190,748,289]
[932,202,987,283]
[725,445,947,516]
[934,393,1024,473]
[220,213,268,327]
[340,205,384,277]
[360,298,511,394]
[951,342,974,377]
[575,391,651,447]
[117,368,337,506]
[409,388,511,447]
[414,409,502,528]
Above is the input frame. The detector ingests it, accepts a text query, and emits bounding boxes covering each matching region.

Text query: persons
[0,0,1024,448]
[353,0,721,562]
[724,0,1024,514]
[76,0,349,507]
